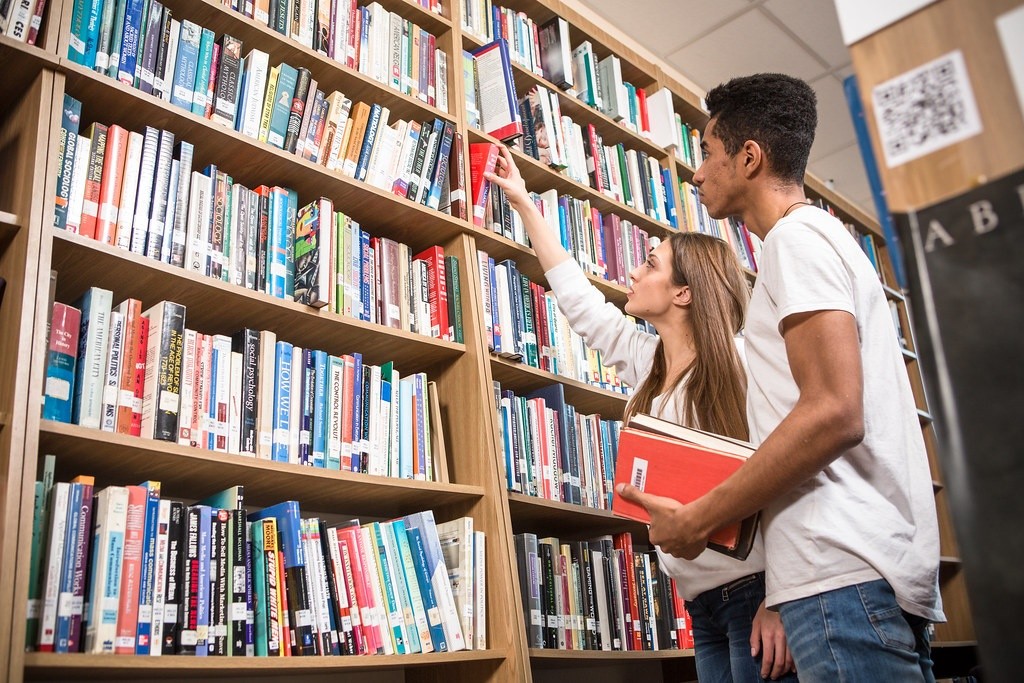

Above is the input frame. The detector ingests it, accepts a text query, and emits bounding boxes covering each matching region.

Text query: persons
[691,72,951,683]
[484,139,800,683]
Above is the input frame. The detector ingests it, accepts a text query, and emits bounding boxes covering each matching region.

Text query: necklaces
[782,202,809,217]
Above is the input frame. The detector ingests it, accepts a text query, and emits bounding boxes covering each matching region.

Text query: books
[509,531,697,653]
[808,198,907,351]
[473,246,658,396]
[0,0,449,116]
[490,379,624,513]
[72,0,467,220]
[461,38,764,289]
[461,0,706,172]
[25,455,487,659]
[611,411,761,561]
[42,269,451,484]
[53,92,464,345]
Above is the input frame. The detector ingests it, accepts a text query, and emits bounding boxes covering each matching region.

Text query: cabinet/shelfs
[0,0,979,683]
